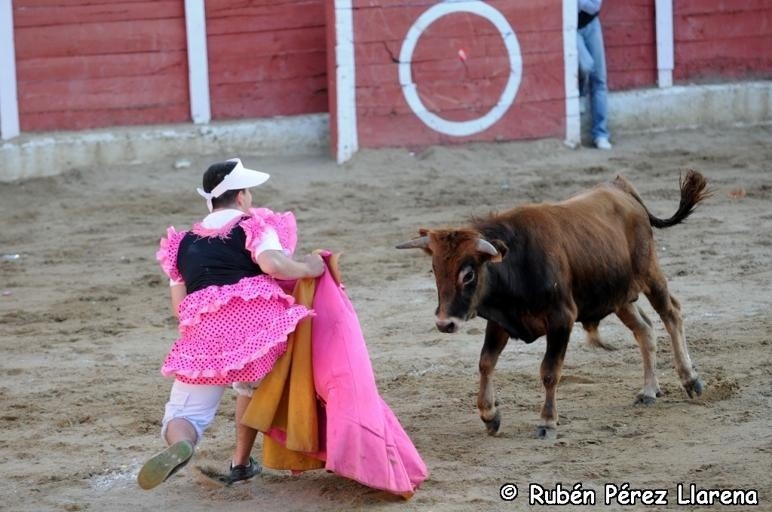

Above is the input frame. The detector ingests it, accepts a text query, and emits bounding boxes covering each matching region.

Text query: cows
[394,166,723,441]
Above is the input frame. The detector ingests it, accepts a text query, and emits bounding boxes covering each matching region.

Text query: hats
[195,158,272,199]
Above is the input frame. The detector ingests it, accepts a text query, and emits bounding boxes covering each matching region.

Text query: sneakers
[228,455,264,486]
[137,440,194,490]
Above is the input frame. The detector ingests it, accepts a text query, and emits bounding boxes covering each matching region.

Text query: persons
[577,0,614,152]
[138,155,327,491]
